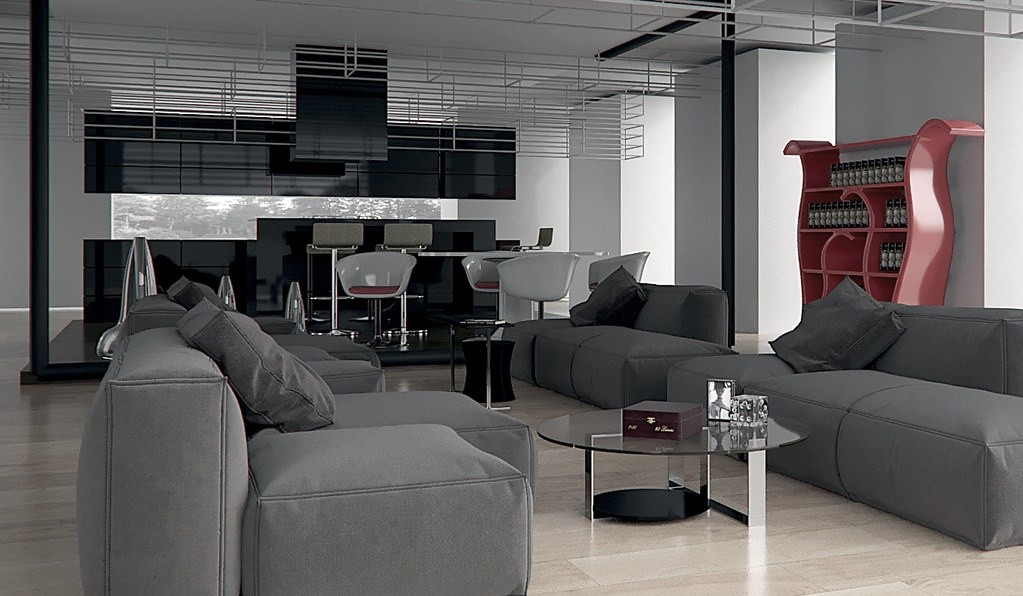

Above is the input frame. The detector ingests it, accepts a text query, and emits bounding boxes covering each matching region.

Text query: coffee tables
[450,321,516,411]
[537,408,812,552]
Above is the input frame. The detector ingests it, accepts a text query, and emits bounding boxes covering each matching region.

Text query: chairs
[336,250,418,349]
[460,251,651,319]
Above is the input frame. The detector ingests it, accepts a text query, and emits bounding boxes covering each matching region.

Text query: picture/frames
[705,378,736,423]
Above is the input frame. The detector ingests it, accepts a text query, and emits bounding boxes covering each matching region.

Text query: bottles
[807,156,907,273]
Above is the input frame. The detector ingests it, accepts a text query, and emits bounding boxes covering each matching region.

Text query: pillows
[768,276,907,374]
[167,276,336,433]
[569,264,648,327]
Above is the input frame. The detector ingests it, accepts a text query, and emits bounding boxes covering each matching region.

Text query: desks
[417,249,610,257]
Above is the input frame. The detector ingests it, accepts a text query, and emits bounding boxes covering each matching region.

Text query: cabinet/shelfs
[783,119,986,304]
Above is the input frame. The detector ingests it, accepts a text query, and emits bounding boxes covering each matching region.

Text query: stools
[307,222,435,337]
[503,228,554,319]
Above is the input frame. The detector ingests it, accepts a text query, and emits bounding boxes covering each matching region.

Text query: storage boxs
[623,399,705,440]
[730,394,769,427]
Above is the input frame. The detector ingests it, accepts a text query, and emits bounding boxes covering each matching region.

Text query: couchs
[501,282,740,411]
[667,301,1023,550]
[73,293,537,596]
